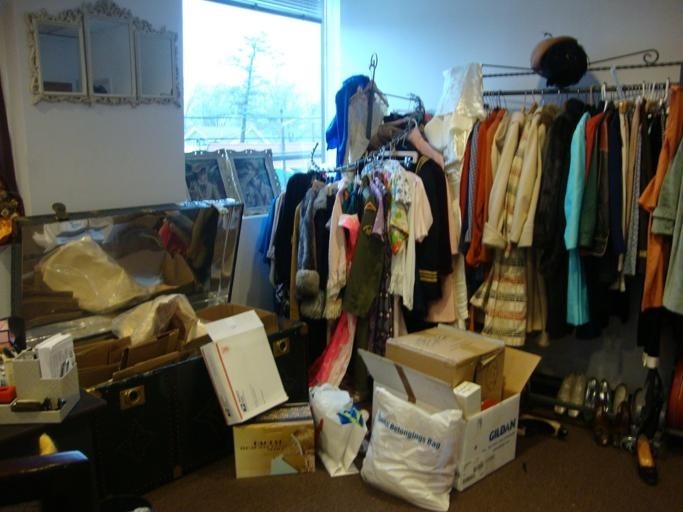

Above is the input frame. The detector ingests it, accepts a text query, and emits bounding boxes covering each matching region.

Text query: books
[37,332,76,380]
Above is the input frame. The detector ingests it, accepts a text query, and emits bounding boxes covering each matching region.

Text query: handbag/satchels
[306,382,370,478]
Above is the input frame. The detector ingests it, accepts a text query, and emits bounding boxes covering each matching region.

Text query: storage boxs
[232,402,316,478]
[385,326,504,402]
[202,309,288,425]
[359,347,538,492]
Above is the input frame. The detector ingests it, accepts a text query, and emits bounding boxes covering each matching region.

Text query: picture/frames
[183,151,283,216]
[26,1,181,108]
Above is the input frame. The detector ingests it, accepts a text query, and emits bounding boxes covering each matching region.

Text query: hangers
[316,120,417,200]
[468,56,681,107]
[348,54,412,145]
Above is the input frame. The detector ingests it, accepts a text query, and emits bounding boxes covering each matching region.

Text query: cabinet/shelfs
[0,318,196,492]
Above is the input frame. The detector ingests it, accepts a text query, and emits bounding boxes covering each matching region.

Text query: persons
[249,174,274,205]
[244,157,258,207]
[188,163,222,202]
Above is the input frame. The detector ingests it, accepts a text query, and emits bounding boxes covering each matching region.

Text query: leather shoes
[633,431,659,486]
[517,413,569,438]
[554,372,662,452]
